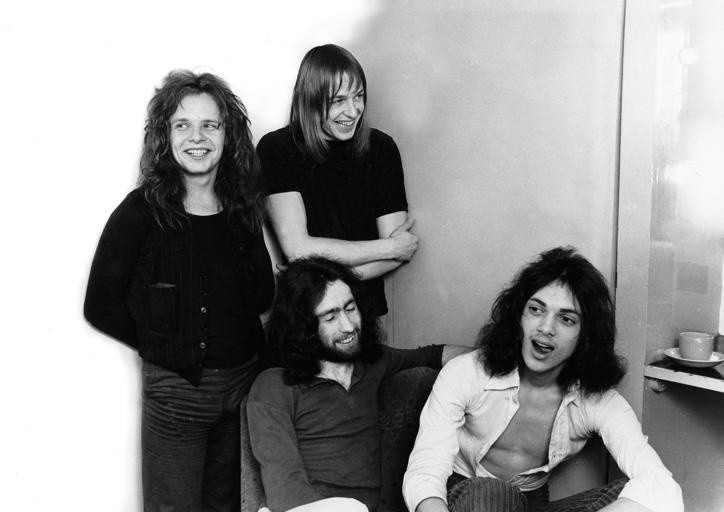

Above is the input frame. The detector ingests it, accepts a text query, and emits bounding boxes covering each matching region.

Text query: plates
[664,345,724,368]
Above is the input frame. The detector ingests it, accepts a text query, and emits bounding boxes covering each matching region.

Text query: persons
[249,258,482,512]
[398,247,685,512]
[82,64,273,512]
[254,43,418,346]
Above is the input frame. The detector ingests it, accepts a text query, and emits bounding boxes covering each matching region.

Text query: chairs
[235,365,438,512]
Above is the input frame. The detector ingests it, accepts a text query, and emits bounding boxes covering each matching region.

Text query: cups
[678,330,714,359]
